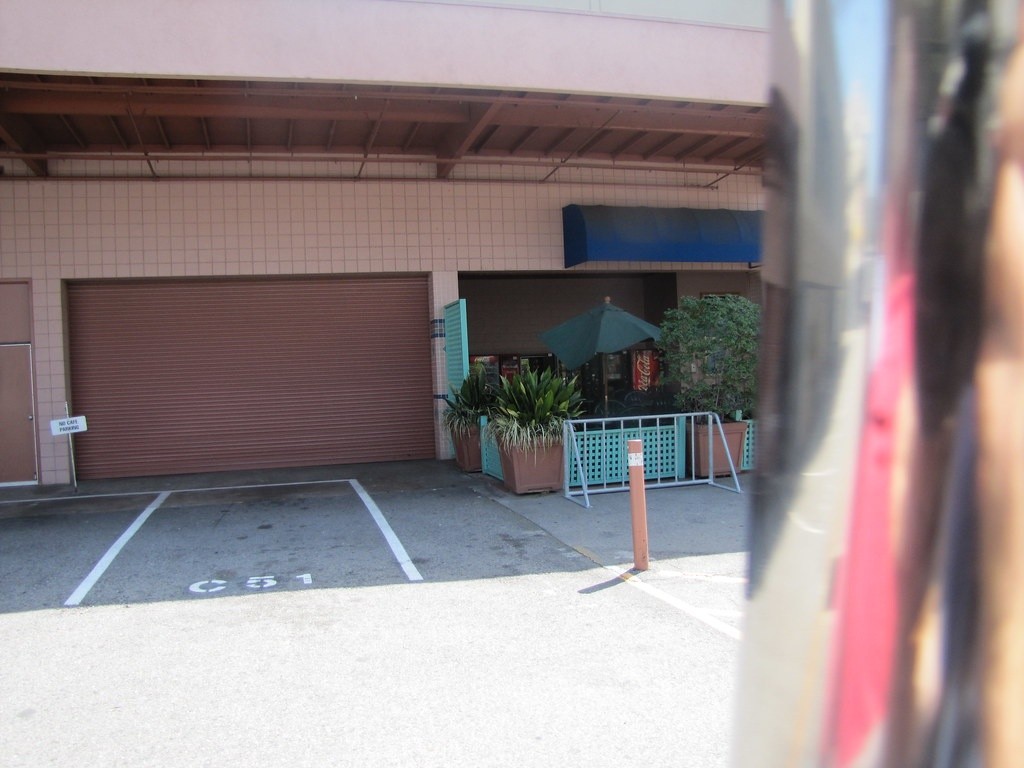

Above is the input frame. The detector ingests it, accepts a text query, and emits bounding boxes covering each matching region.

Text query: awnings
[563,203,767,268]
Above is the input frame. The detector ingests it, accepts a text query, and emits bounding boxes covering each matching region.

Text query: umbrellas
[540,303,664,403]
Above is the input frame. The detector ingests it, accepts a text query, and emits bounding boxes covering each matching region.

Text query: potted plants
[482,362,587,495]
[441,359,501,473]
[654,294,763,478]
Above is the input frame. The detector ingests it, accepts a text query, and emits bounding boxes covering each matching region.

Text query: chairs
[573,391,671,430]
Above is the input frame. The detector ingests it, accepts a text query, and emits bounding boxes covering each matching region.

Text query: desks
[576,414,614,424]
